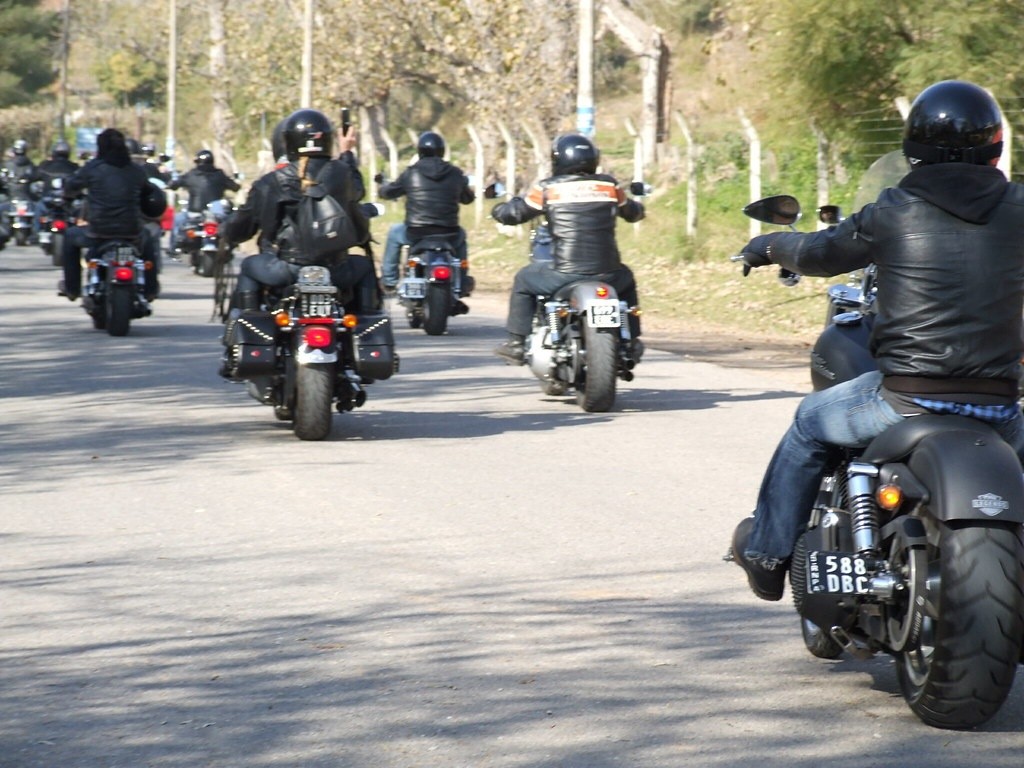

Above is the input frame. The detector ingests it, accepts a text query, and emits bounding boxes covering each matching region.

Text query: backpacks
[283,160,360,262]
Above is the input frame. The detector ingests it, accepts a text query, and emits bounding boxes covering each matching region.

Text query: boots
[357,288,377,317]
[234,290,260,309]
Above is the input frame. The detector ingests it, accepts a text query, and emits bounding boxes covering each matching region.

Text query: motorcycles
[723,194,1023,729]
[368,170,479,336]
[166,171,246,279]
[48,172,152,339]
[0,165,83,267]
[482,181,651,413]
[207,196,400,443]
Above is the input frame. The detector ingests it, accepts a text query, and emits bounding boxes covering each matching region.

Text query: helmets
[50,142,69,159]
[551,135,597,177]
[12,139,28,157]
[418,131,445,161]
[902,79,1002,170]
[271,118,291,162]
[196,150,214,171]
[142,193,168,219]
[125,137,142,156]
[288,109,331,157]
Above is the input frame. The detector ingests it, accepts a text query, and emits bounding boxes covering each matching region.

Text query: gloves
[741,234,776,277]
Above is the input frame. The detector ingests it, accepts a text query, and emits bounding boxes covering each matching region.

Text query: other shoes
[145,283,158,300]
[629,336,643,365]
[378,276,398,297]
[733,517,789,600]
[460,277,473,298]
[59,279,77,301]
[494,341,523,367]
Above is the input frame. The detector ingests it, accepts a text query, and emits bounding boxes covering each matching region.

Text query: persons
[56,128,161,301]
[0,139,80,243]
[732,79,1024,601]
[218,108,383,380]
[167,150,241,257]
[125,139,171,184]
[378,132,475,294]
[492,134,646,366]
